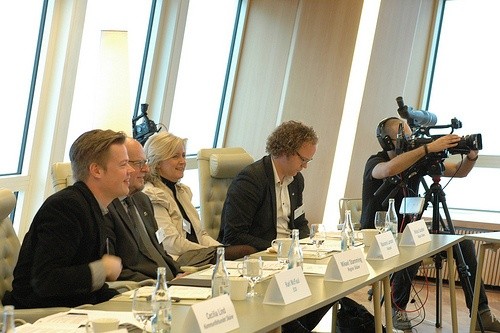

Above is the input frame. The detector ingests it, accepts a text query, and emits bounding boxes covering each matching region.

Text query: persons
[107,138,190,288]
[141,132,258,274]
[1,129,133,309]
[215,119,337,333]
[358,117,500,333]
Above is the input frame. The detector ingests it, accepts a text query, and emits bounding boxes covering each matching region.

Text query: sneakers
[392,308,412,333]
[475,308,500,333]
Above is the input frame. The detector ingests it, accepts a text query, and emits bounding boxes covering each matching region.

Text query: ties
[123,196,174,281]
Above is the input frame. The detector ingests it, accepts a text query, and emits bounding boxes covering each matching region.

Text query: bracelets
[467,155,478,161]
[424,144,428,154]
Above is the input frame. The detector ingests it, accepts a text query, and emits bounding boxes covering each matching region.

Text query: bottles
[341,210,355,253]
[288,229,303,271]
[212,247,229,299]
[385,199,398,244]
[152,267,173,333]
[2,306,15,333]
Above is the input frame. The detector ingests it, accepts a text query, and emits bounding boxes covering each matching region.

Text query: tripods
[381,174,485,333]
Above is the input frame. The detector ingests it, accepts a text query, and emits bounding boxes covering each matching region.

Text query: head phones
[376,119,395,151]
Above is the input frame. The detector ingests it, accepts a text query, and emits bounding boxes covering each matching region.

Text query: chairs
[339,198,443,328]
[0,148,254,333]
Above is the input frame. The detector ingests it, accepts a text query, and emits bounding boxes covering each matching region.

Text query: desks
[84,232,500,333]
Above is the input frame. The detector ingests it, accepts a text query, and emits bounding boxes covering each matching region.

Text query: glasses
[129,159,149,168]
[297,151,313,164]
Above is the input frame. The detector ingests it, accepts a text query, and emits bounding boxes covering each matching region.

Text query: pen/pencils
[299,243,313,245]
[68,313,87,315]
[154,299,179,302]
[106,238,109,255]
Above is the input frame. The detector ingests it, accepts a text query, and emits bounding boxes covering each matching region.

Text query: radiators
[417,225,500,289]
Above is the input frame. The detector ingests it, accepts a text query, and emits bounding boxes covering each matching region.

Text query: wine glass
[244,256,263,297]
[375,212,390,234]
[132,287,154,333]
[277,242,292,271]
[310,224,325,257]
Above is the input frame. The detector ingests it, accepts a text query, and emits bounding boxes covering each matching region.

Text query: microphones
[411,109,437,125]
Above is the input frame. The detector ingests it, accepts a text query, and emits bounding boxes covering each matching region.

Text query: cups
[237,261,264,276]
[227,277,248,300]
[356,229,379,247]
[272,238,294,255]
[86,318,119,333]
